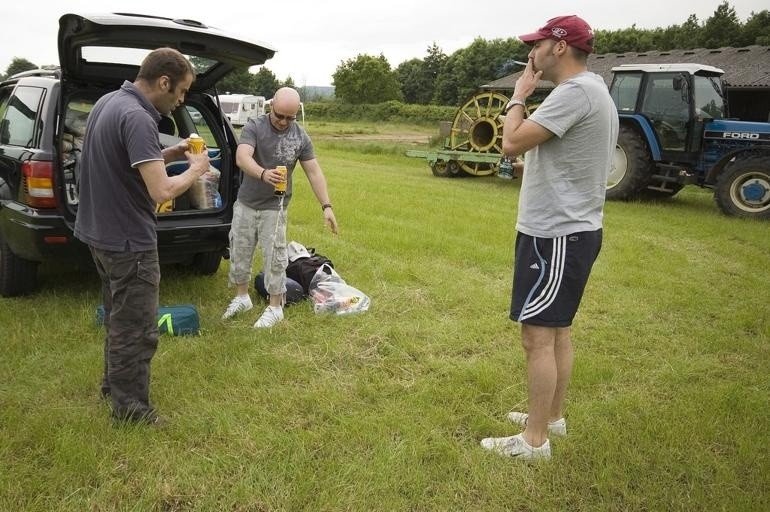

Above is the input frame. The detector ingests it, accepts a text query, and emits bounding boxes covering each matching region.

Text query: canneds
[497,153,517,178]
[275,166,287,196]
[189,137,205,154]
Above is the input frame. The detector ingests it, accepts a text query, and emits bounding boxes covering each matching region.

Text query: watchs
[507,101,526,110]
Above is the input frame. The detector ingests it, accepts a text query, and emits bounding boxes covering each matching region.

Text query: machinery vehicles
[601,61,770,221]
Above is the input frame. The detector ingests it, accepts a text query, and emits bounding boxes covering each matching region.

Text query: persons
[73,47,210,426]
[479,16,620,462]
[222,88,338,327]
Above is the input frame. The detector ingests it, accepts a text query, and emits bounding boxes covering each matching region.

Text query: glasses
[272,107,297,123]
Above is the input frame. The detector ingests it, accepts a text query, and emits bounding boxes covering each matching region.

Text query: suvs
[0,8,282,301]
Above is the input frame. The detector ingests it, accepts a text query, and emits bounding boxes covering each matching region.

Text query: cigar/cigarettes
[512,59,527,66]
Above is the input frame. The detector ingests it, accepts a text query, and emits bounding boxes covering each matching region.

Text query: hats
[287,241,311,263]
[518,13,594,55]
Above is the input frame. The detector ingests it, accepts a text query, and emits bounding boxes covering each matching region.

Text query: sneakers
[506,411,567,438]
[222,295,254,320]
[479,433,551,461]
[254,306,284,329]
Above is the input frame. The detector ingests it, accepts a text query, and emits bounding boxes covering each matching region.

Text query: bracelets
[261,169,268,185]
[322,204,332,211]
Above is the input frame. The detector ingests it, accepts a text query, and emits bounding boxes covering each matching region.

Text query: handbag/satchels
[95,304,201,338]
[255,271,305,307]
[285,247,334,296]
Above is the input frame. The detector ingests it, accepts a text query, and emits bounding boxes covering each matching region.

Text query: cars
[188,109,208,127]
